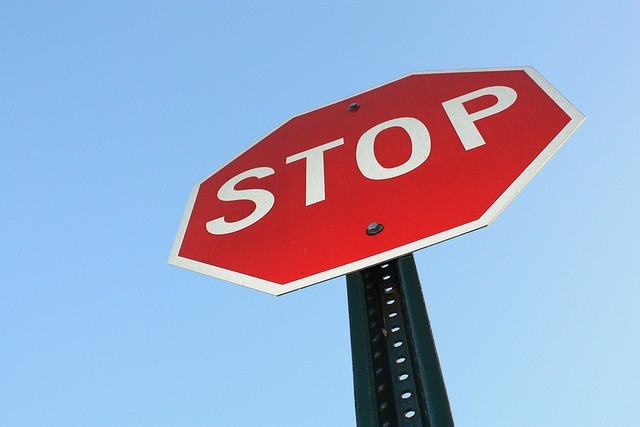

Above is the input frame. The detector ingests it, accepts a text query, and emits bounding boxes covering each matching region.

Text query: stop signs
[168,66,587,297]
